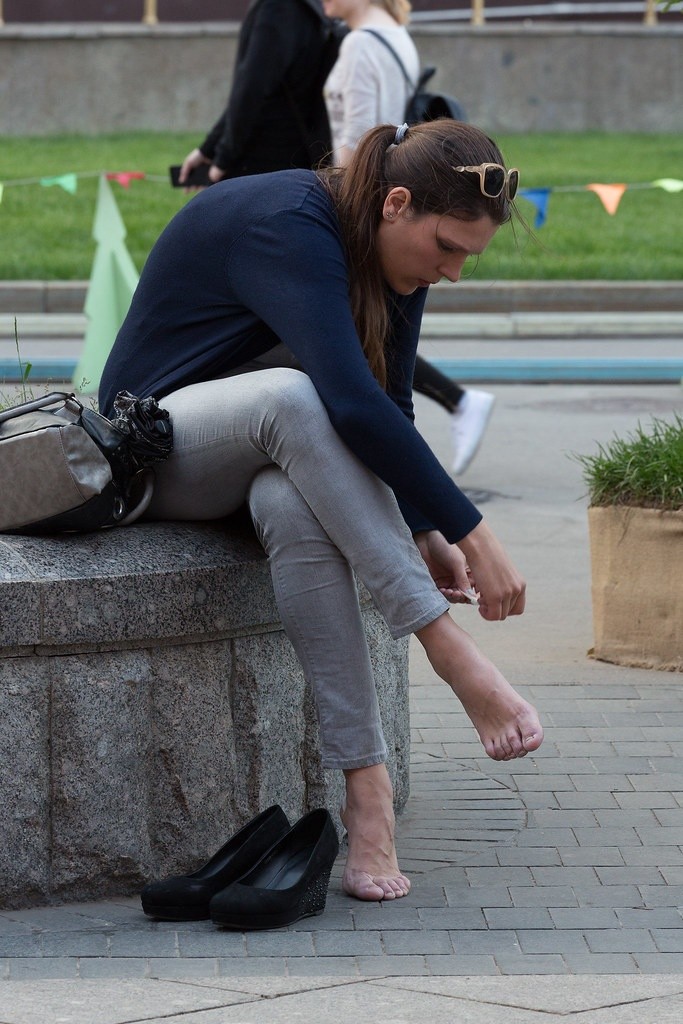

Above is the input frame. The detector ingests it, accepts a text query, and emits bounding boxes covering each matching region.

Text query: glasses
[450,162,520,202]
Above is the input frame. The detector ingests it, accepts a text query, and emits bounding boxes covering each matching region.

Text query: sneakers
[449,389,496,477]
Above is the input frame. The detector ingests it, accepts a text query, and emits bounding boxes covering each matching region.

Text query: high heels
[210,808,339,929]
[139,804,291,921]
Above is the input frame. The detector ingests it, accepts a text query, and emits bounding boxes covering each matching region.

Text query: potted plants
[564,413,683,673]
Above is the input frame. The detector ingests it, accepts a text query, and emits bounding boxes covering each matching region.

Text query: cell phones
[170,163,211,187]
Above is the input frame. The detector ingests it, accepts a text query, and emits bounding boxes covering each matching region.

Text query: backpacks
[361,28,466,126]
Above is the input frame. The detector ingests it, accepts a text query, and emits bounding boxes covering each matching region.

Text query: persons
[176,0,333,194]
[322,0,497,477]
[96,119,544,900]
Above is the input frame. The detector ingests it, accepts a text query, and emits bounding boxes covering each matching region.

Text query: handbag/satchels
[0,392,154,534]
